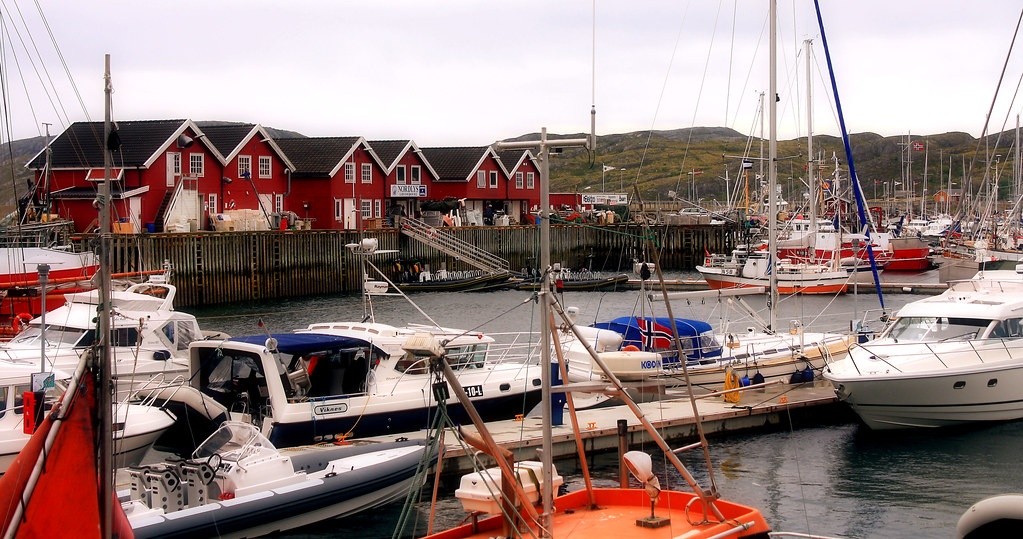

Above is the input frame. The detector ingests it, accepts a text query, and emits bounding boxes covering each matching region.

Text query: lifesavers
[12,313,34,335]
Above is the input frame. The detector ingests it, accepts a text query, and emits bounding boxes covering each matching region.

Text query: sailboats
[0,0,773,539]
[0,0,179,340]
[529,0,1023,429]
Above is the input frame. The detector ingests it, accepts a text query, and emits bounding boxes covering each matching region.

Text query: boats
[0,257,226,484]
[516,268,630,294]
[395,261,510,293]
[140,144,553,461]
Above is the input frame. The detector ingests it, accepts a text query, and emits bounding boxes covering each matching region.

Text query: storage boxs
[113,222,134,234]
